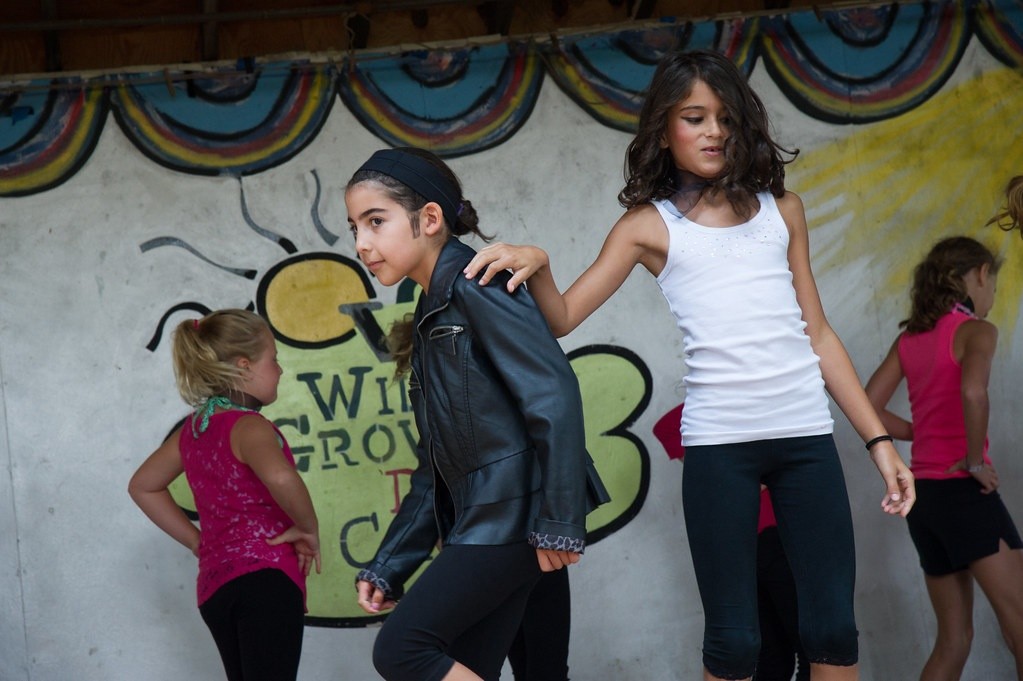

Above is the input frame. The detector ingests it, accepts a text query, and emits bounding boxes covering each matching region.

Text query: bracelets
[962,454,985,472]
[865,435,894,450]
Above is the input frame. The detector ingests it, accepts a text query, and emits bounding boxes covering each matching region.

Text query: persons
[388,320,570,681]
[344,149,611,681]
[865,236,1023,681]
[755,485,811,681]
[463,52,915,681]
[127,309,323,681]
